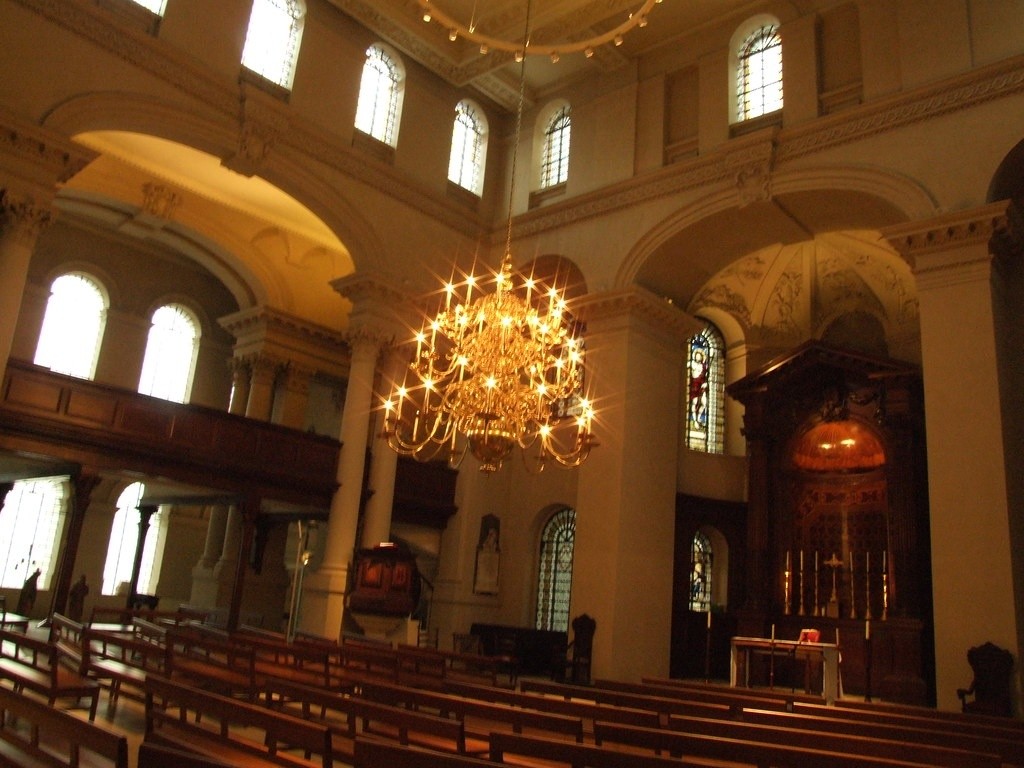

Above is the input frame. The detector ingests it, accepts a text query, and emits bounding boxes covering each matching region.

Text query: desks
[730,636,840,699]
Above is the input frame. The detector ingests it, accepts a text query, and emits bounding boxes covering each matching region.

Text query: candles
[836,628,839,646]
[707,609,711,628]
[782,545,888,573]
[772,624,774,643]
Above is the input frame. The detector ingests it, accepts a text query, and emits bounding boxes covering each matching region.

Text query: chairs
[417,625,439,651]
[452,632,485,678]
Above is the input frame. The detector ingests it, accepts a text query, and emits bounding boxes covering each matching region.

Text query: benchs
[0,595,1024,768]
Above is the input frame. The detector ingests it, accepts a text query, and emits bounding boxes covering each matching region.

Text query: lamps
[789,404,886,474]
[376,1,664,477]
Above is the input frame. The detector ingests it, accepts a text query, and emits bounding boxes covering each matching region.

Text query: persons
[69,576,88,623]
[16,570,40,619]
